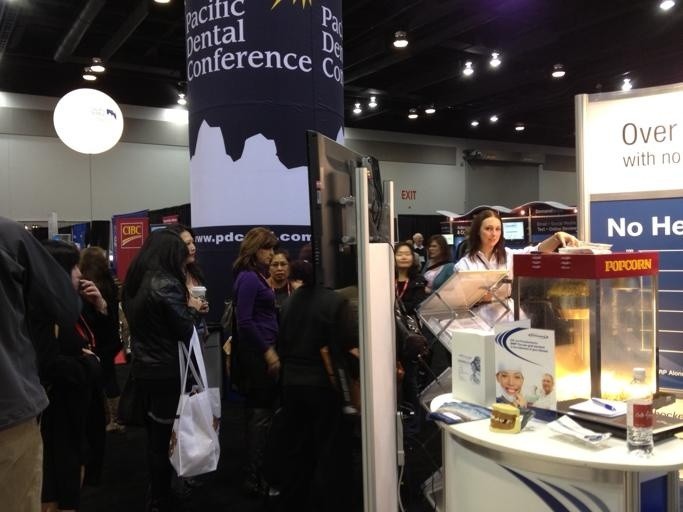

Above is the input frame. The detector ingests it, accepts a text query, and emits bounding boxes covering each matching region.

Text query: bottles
[626,367,655,458]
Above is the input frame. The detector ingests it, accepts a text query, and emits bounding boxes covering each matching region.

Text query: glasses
[270,262,289,266]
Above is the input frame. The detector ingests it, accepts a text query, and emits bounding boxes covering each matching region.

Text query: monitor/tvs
[501,220,527,243]
[304,128,383,291]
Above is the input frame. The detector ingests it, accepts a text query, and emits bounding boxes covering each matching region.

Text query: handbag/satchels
[168,324,222,478]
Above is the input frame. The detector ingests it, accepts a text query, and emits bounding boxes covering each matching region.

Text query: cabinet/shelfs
[512,250,661,402]
[430,391,683,512]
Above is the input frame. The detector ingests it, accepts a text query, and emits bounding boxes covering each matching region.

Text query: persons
[82,247,127,432]
[116,229,195,507]
[41,241,114,508]
[452,210,577,278]
[0,217,84,508]
[266,249,297,303]
[412,233,427,263]
[167,223,209,322]
[395,242,430,316]
[534,373,556,411]
[495,360,525,410]
[232,229,281,499]
[424,233,455,292]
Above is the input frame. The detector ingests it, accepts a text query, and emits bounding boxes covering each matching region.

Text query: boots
[240,409,280,497]
[105,397,125,434]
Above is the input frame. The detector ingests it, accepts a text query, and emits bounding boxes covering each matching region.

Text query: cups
[192,287,207,300]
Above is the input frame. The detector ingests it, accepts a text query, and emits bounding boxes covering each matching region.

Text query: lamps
[89,57,106,74]
[351,102,362,115]
[425,102,436,114]
[489,51,502,68]
[176,91,186,106]
[551,63,565,79]
[621,76,633,92]
[392,29,410,50]
[462,58,474,76]
[407,108,418,121]
[369,95,378,109]
[82,67,97,83]
[515,119,526,131]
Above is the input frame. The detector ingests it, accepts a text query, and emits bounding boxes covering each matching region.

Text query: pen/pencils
[591,397,617,412]
